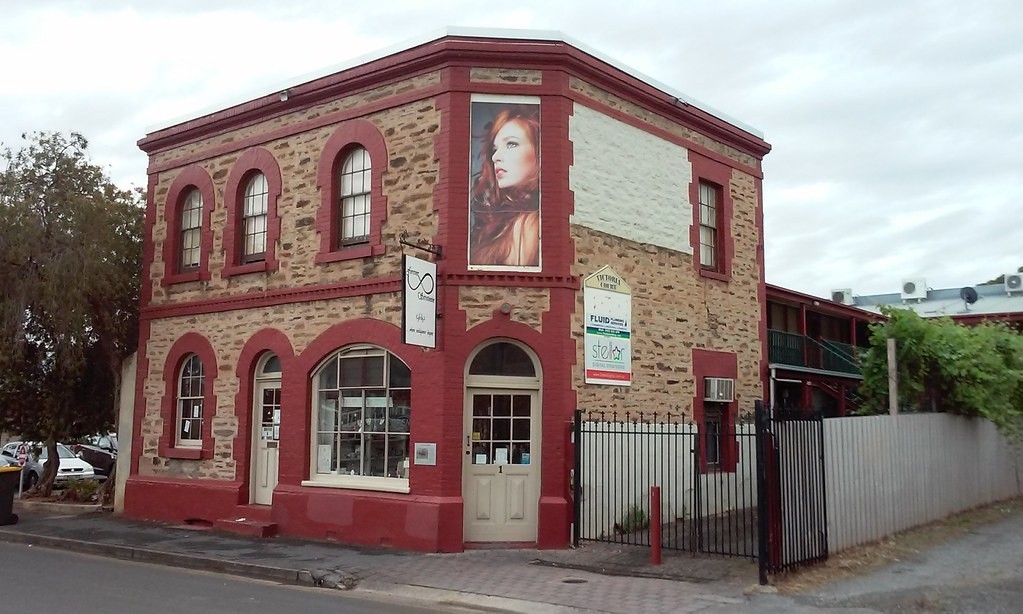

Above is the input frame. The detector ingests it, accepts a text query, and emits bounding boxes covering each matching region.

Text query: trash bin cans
[0,466,24,526]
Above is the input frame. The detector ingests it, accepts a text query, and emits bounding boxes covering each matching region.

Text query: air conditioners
[1004,272,1023,292]
[831,289,852,305]
[900,278,927,299]
[703,378,734,402]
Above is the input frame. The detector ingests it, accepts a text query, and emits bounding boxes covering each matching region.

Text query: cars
[70,431,118,479]
[0,440,96,494]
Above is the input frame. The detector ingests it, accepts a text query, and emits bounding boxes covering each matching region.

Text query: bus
[341,405,410,433]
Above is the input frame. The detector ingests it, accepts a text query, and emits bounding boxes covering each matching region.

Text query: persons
[470,104,539,266]
[513,444,523,464]
